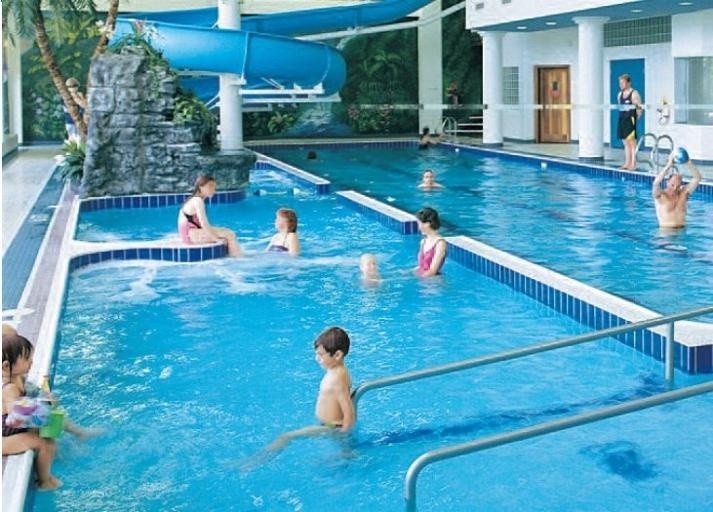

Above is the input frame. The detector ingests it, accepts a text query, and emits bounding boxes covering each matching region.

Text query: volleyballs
[672,148,688,164]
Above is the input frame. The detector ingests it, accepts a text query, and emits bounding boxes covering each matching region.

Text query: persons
[256,208,298,254]
[403,207,449,279]
[176,175,241,257]
[360,253,380,281]
[652,149,702,234]
[256,325,359,460]
[417,127,446,151]
[417,169,444,191]
[1,325,110,441]
[1,334,61,489]
[616,73,642,172]
